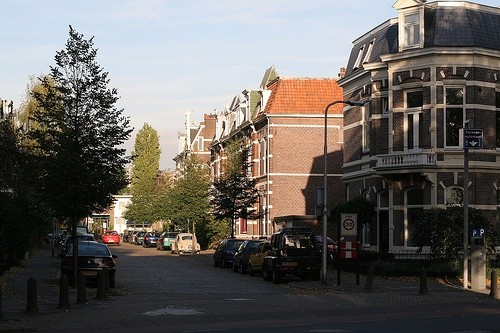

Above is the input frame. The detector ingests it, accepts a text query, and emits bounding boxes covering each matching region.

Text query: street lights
[322,100,365,284]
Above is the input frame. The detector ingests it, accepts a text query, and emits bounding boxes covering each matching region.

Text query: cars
[63,234,98,253]
[61,242,118,288]
[232,239,267,274]
[123,230,138,245]
[102,230,121,246]
[314,235,338,256]
[156,232,180,251]
[212,239,247,269]
[174,233,200,254]
[134,231,148,246]
[142,232,160,248]
[247,241,271,275]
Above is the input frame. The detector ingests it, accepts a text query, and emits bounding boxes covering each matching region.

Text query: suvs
[263,227,321,284]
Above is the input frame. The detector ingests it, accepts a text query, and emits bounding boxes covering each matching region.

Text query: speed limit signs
[340,212,359,236]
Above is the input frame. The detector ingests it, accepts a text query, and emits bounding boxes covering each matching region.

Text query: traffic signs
[461,128,484,150]
